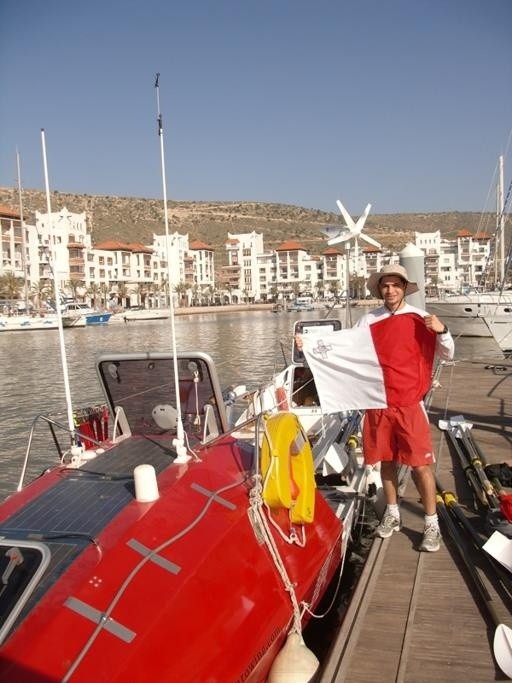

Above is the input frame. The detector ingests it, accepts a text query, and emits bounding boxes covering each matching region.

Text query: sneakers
[418,525,440,552]
[373,514,403,538]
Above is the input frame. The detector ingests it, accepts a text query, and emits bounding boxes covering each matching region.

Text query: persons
[295,275,455,552]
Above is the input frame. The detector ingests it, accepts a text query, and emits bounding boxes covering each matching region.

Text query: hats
[366,265,420,299]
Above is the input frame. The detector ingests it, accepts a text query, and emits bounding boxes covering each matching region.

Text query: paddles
[322,411,363,476]
[432,416,512,677]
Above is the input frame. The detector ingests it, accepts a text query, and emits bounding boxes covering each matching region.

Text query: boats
[0,71,387,681]
[323,302,358,308]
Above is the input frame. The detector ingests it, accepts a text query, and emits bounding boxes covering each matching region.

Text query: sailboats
[0,144,171,331]
[427,154,512,351]
